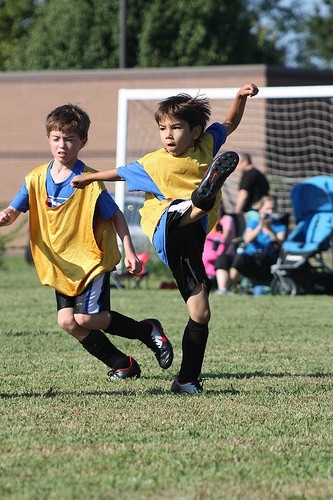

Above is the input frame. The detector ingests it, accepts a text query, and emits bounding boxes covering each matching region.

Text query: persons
[0,104,173,380]
[202,153,287,296]
[70,83,259,394]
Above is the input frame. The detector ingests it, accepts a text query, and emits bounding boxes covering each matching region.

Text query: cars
[106,192,156,273]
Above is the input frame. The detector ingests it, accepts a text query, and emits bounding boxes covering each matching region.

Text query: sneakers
[106,355,141,381]
[170,375,206,396]
[140,318,174,369]
[194,150,240,202]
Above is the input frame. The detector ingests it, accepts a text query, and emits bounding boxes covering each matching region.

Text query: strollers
[270,176,333,296]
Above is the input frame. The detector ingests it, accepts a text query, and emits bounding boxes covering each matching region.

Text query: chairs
[227,212,289,293]
[107,253,149,289]
